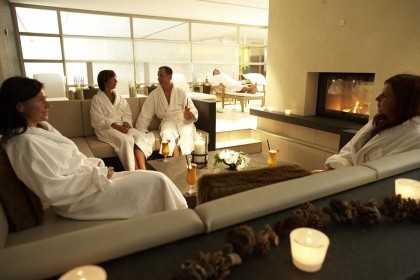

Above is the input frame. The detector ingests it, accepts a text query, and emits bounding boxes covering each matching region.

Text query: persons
[135,66,198,156]
[90,70,155,171]
[211,69,257,94]
[0,76,188,219]
[325,74,420,169]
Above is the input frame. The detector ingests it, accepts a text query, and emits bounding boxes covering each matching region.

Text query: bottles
[74,76,77,85]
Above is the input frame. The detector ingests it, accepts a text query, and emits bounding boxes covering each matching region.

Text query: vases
[223,161,238,170]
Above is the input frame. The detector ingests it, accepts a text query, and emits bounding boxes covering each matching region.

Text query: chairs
[32,73,69,100]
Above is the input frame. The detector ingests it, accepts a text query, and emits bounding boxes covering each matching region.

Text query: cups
[66,87,84,100]
[394,178,420,206]
[58,265,107,280]
[129,85,137,97]
[289,227,330,273]
[267,149,278,167]
[284,108,290,115]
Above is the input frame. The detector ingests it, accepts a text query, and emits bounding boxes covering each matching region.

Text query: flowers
[213,145,247,168]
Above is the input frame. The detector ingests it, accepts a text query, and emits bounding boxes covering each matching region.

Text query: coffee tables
[140,146,292,206]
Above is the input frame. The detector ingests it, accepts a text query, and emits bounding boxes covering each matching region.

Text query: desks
[215,91,264,114]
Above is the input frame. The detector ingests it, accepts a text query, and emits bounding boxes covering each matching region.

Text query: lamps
[285,109,291,116]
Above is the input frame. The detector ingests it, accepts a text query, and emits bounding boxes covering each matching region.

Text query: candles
[59,265,107,280]
[289,226,330,273]
[394,177,420,205]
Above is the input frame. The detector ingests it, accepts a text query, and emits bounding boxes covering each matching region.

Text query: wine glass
[161,140,170,163]
[186,163,196,194]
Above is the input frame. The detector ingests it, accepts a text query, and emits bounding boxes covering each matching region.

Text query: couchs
[0,93,420,280]
